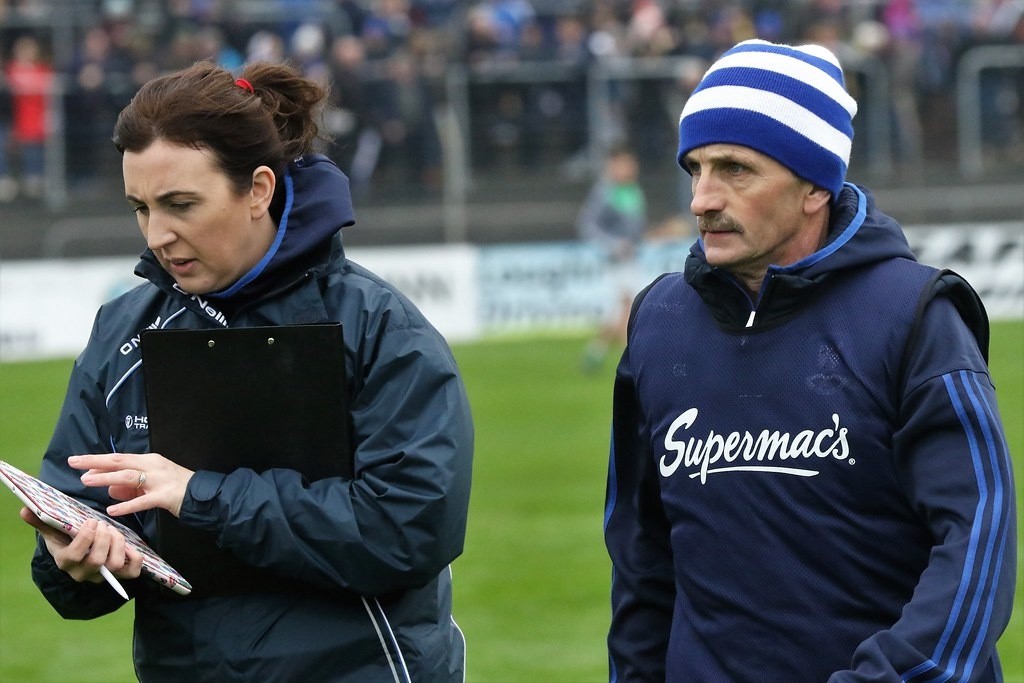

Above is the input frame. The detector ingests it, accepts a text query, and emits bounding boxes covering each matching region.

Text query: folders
[139,319,358,597]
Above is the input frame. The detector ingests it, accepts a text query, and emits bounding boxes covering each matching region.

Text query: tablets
[0,459,193,596]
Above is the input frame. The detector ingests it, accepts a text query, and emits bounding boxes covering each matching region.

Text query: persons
[604,38,1018,683]
[575,141,647,364]
[0,1,1023,193]
[21,58,476,683]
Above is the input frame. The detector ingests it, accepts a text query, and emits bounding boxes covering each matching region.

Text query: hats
[675,38,861,204]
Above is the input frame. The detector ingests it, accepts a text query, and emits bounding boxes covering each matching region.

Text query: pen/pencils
[77,543,130,601]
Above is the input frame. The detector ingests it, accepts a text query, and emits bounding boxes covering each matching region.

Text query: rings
[136,471,145,489]
